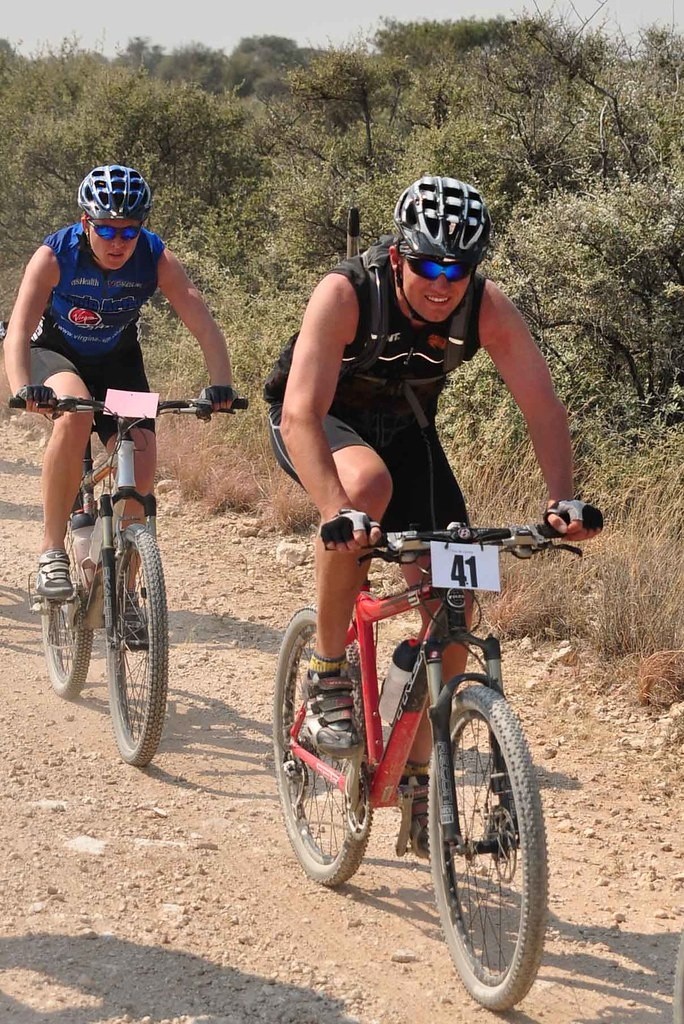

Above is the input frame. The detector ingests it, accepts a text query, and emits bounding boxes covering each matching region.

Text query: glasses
[87,220,143,240]
[401,253,476,281]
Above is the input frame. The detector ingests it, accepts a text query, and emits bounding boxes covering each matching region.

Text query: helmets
[393,176,492,264]
[78,165,151,220]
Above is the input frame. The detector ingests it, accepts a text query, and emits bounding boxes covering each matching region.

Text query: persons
[259,177,605,859]
[3,165,237,652]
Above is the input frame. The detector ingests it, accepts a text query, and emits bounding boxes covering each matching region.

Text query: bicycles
[273,500,590,1011]
[7,381,250,770]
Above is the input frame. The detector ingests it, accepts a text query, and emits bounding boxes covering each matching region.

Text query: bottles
[379,638,419,722]
[71,509,95,586]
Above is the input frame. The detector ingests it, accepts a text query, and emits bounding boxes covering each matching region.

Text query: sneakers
[303,670,359,757]
[36,548,73,598]
[397,774,430,858]
[117,592,148,649]
